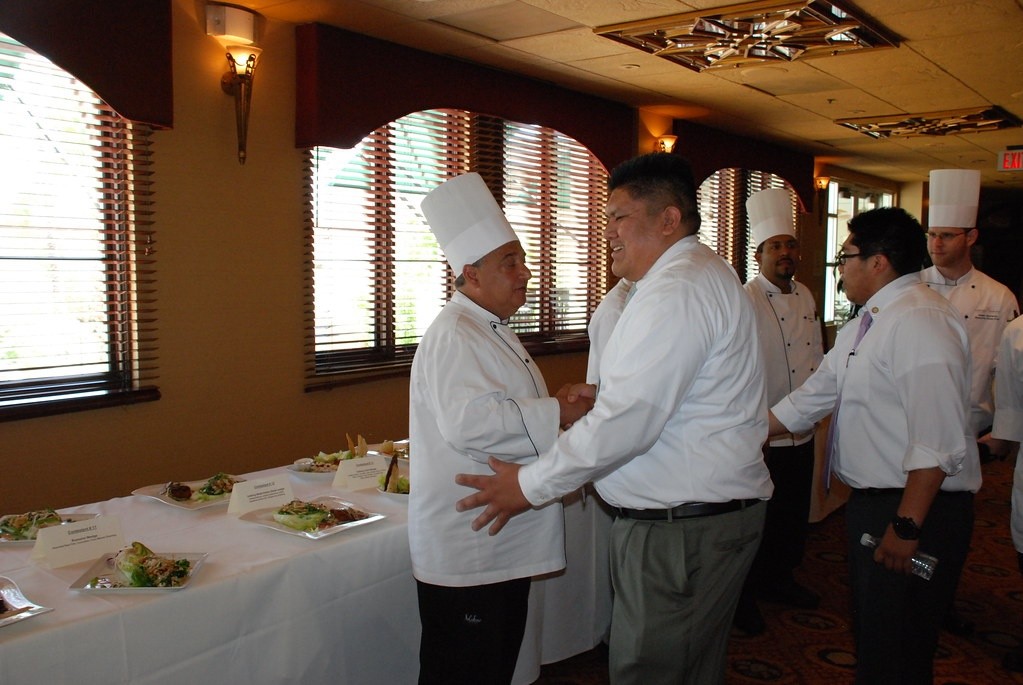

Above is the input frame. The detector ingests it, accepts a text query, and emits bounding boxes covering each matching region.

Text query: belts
[615,496,758,520]
[850,487,960,501]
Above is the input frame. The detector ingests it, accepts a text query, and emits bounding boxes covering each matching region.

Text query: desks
[0,399,854,685]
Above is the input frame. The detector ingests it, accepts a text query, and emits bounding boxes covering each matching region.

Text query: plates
[0,576,56,630]
[285,464,336,482]
[376,486,409,504]
[354,442,409,464]
[67,551,209,591]
[0,512,101,547]
[235,495,387,540]
[131,472,250,511]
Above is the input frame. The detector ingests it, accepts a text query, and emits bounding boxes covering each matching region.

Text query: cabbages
[116,553,156,588]
[274,513,328,530]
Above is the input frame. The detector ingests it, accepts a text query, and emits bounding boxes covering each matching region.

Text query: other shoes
[941,605,975,634]
[1003,645,1023,672]
[773,570,822,610]
[732,573,774,635]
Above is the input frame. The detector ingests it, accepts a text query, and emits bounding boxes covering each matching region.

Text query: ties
[823,311,873,497]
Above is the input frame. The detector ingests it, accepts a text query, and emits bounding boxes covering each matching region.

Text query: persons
[455,151,776,685]
[737,188,826,624]
[586,276,639,388]
[765,206,978,685]
[406,172,595,685]
[920,167,1023,608]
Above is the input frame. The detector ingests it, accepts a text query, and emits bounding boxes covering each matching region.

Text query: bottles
[860,532,938,580]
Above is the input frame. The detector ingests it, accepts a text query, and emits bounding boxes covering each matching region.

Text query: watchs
[892,513,920,541]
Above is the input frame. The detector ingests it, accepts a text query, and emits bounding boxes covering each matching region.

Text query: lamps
[658,134,678,154]
[221,45,263,165]
[816,177,831,225]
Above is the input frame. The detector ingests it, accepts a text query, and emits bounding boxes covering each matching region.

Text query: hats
[928,169,981,228]
[420,171,519,280]
[745,188,797,256]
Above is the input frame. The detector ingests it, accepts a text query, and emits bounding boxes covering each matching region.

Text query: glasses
[923,227,972,241]
[835,250,889,265]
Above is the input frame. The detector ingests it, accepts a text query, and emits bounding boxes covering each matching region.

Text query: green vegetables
[0,510,59,540]
[312,450,355,463]
[378,473,409,495]
[278,500,326,514]
[198,473,231,496]
[149,557,192,588]
